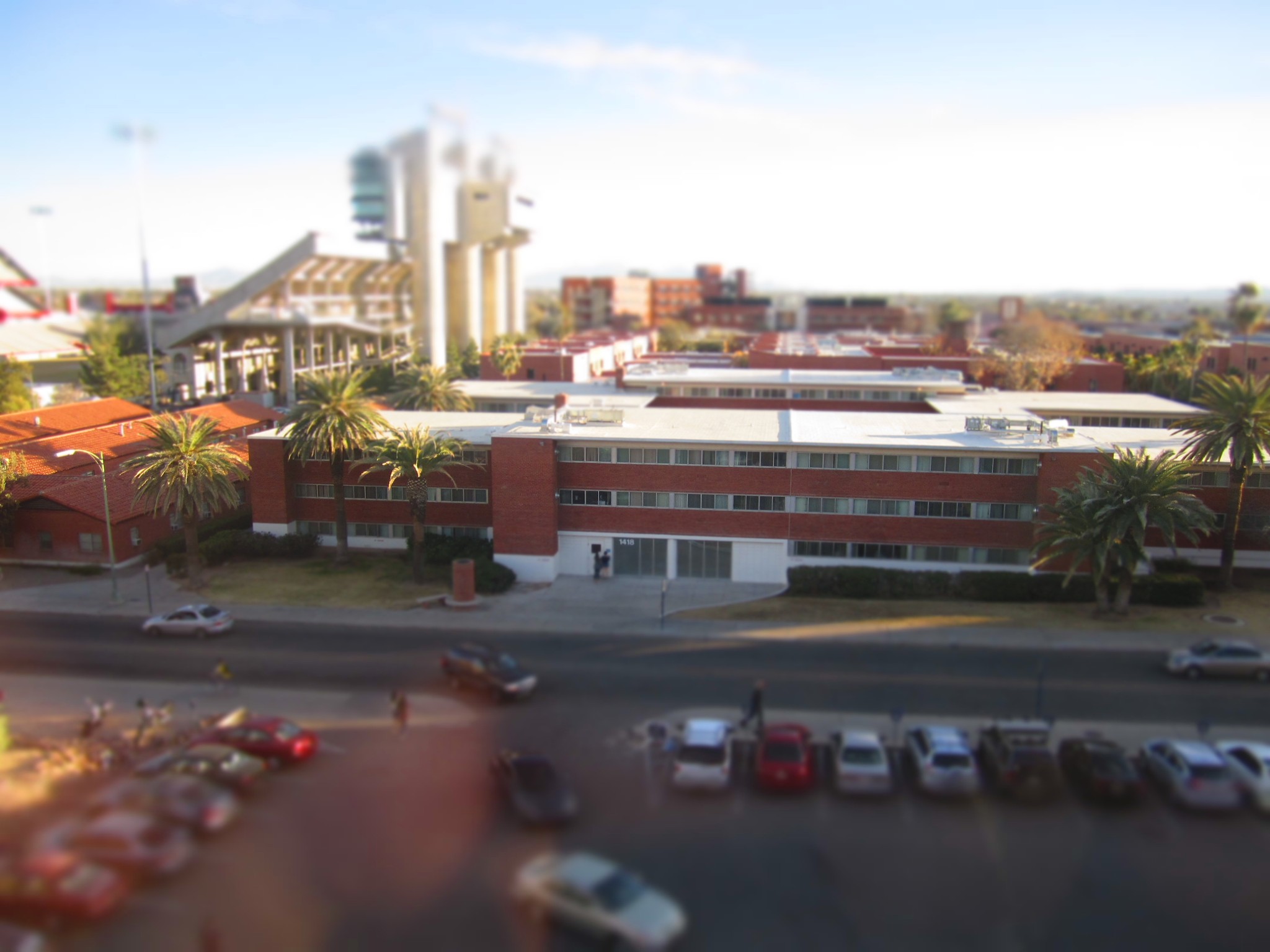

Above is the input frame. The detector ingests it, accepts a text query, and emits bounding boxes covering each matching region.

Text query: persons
[85,695,104,735]
[0,903,58,952]
[738,679,764,735]
[599,551,610,580]
[133,697,165,749]
[392,690,408,735]
[199,927,219,952]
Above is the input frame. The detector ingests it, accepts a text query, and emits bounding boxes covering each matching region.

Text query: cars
[490,750,579,825]
[672,717,734,792]
[441,642,538,701]
[904,727,981,796]
[0,921,48,952]
[980,722,1063,798]
[143,604,234,640]
[1143,738,1242,811]
[516,851,688,952]
[86,775,246,838]
[1164,637,1270,683]
[1217,738,1270,812]
[134,741,271,796]
[829,729,892,798]
[193,716,320,773]
[0,852,127,921]
[40,813,194,880]
[756,722,815,791]
[1057,736,1140,801]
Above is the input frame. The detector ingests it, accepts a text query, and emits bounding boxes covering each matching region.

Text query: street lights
[54,449,116,566]
